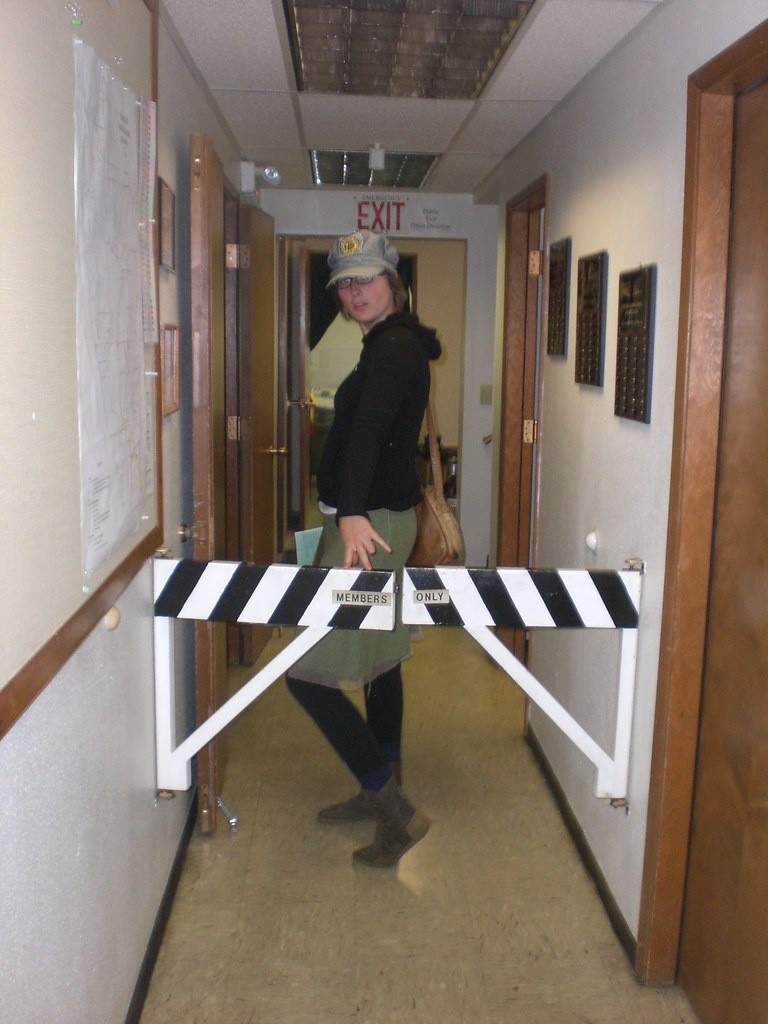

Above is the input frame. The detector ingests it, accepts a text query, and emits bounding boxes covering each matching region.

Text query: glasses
[331,272,386,289]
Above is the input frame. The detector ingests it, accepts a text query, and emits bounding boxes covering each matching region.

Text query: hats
[325,229,400,290]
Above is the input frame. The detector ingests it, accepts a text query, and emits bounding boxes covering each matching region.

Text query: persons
[284,229,442,866]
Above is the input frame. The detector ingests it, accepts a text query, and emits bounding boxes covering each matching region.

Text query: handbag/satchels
[404,486,464,567]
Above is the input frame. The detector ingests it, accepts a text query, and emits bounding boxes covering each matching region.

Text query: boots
[352,775,430,868]
[317,762,402,824]
